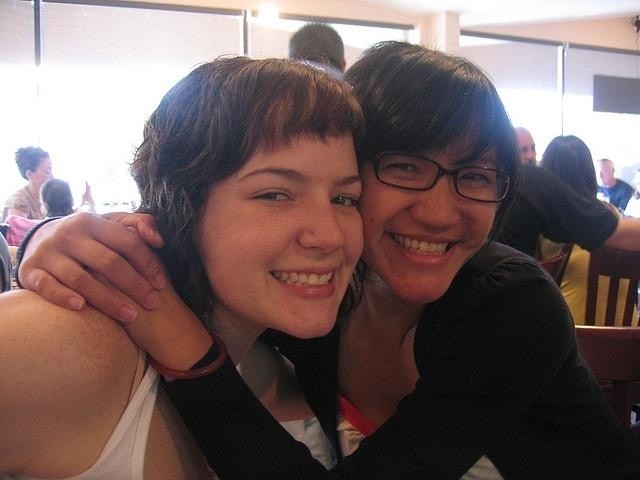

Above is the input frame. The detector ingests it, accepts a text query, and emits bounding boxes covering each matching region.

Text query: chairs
[584,243,640,327]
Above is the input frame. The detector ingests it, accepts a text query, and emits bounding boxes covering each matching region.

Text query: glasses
[370,150,515,202]
